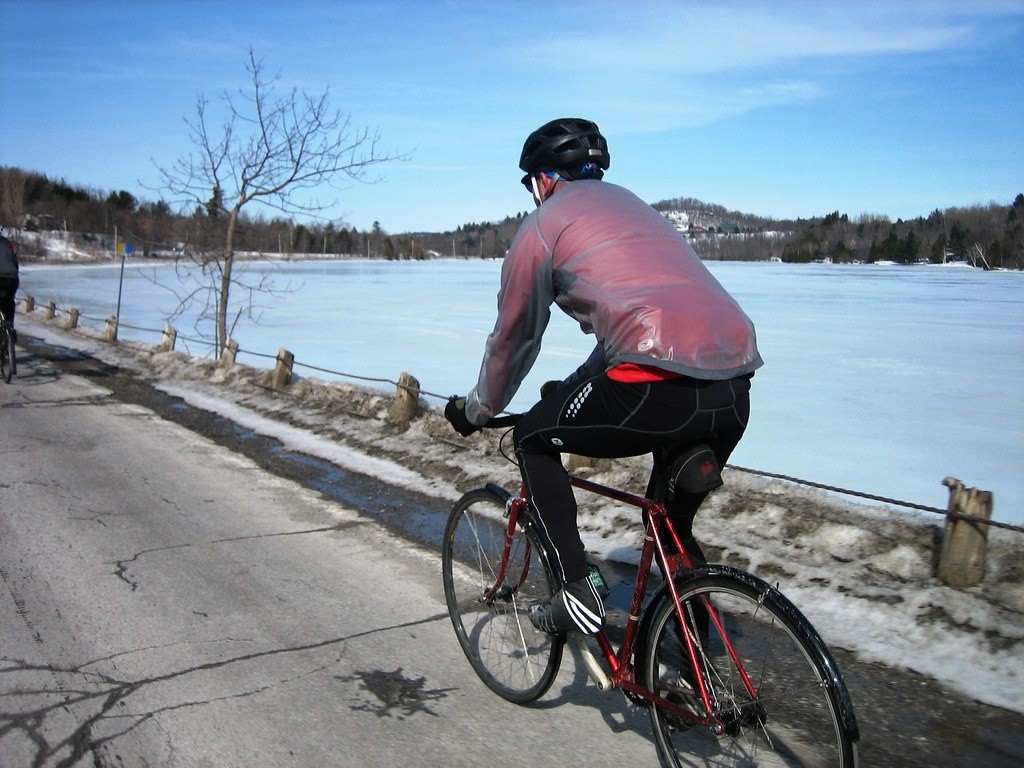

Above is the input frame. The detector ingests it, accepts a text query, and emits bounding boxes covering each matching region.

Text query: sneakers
[658,665,710,709]
[528,576,607,635]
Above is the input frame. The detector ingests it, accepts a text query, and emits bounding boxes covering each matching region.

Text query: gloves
[444,396,482,437]
[541,379,566,397]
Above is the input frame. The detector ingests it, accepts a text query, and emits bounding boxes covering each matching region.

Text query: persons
[443,116,764,681]
[0,234,20,358]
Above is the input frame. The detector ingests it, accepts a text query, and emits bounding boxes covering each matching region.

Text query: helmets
[518,119,610,187]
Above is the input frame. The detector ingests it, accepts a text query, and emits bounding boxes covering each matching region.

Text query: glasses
[521,173,566,192]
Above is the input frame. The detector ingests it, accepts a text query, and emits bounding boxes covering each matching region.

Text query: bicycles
[439,395,863,768]
[0,290,17,383]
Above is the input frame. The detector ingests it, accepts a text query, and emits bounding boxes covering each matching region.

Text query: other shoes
[12,330,17,343]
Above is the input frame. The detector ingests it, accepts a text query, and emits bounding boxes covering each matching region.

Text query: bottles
[584,552,609,600]
